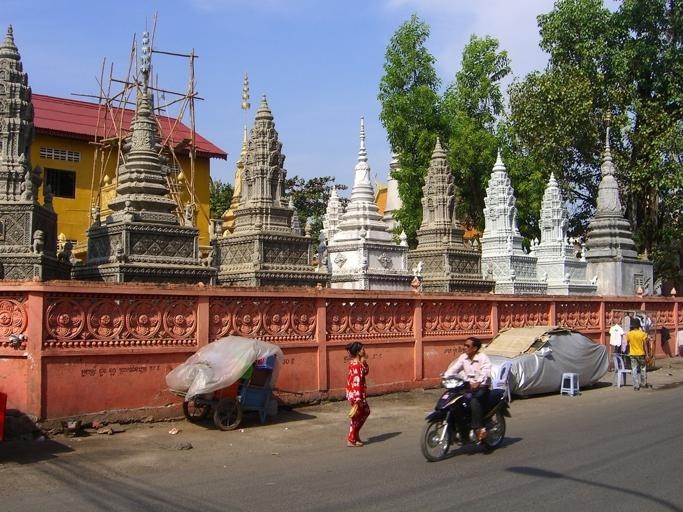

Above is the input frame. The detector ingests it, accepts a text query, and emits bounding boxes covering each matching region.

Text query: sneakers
[347,440,363,446]
[479,429,488,440]
[635,383,647,390]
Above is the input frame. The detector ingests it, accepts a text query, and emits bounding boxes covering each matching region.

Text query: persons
[344,340,371,448]
[626,318,653,391]
[57,242,74,262]
[444,336,494,443]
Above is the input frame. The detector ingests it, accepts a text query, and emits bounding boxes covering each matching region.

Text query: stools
[560,371,580,397]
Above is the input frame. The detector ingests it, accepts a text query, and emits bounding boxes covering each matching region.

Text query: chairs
[610,351,637,389]
[490,361,514,406]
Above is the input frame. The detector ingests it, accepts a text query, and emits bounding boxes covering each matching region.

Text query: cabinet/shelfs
[610,309,655,373]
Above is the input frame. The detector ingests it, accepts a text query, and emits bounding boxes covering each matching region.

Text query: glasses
[464,343,470,348]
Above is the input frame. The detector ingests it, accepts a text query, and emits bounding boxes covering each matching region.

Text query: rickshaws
[170,348,307,429]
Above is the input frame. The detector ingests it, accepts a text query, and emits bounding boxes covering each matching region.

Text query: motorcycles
[418,371,513,462]
